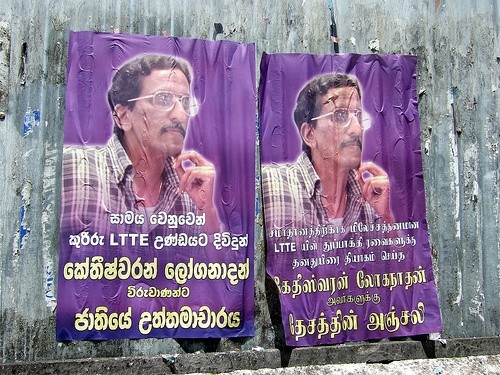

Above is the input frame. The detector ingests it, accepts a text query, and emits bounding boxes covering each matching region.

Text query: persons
[61,50,230,287]
[260,70,412,274]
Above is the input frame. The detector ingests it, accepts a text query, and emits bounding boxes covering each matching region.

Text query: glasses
[310,107,372,132]
[124,91,200,117]
